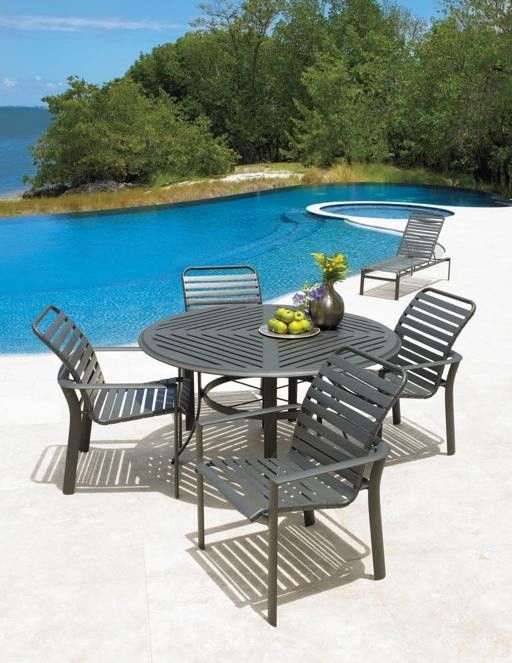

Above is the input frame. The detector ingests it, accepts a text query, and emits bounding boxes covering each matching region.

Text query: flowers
[292,250,347,310]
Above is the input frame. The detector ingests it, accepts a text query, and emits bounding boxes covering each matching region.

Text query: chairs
[183,265,266,430]
[31,306,188,500]
[359,212,450,300]
[194,345,409,627]
[377,289,477,456]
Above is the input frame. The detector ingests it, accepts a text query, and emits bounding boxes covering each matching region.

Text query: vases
[308,278,344,332]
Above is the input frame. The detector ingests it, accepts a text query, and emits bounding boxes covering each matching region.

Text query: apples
[267,308,312,334]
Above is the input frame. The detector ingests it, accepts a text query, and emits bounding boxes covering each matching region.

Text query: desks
[137,304,402,515]
